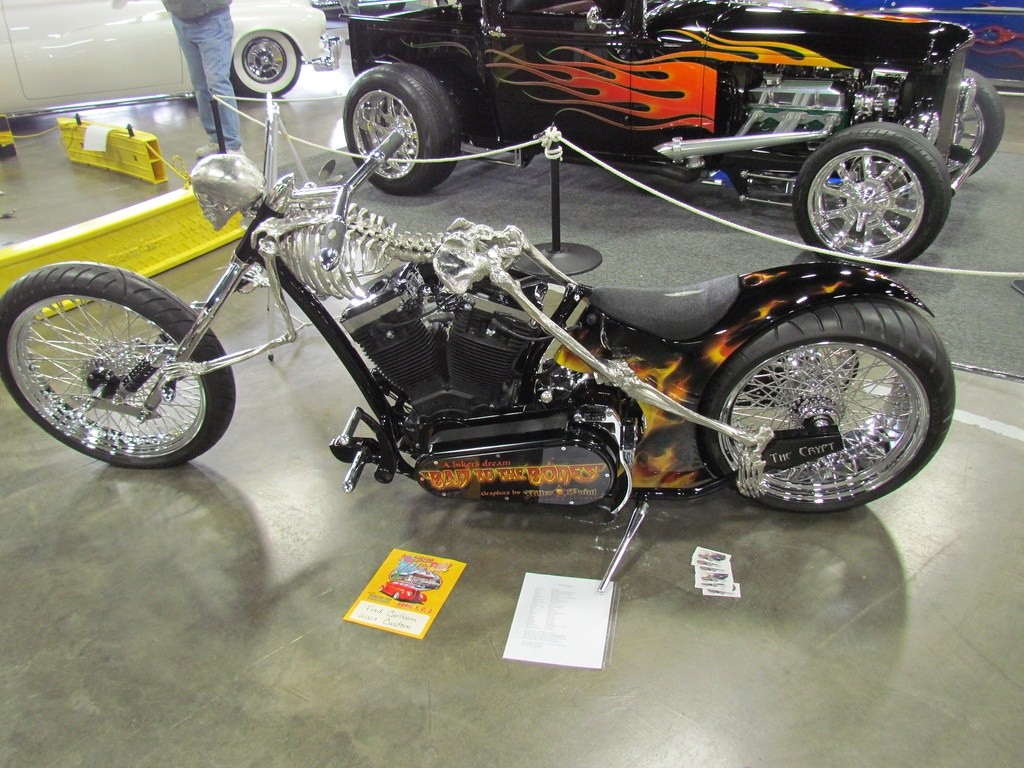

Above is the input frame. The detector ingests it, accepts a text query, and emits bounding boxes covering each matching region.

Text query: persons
[161,0,246,157]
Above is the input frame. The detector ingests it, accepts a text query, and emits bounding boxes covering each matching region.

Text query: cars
[754,1,1024,98]
[335,0,1006,271]
[2,1,341,121]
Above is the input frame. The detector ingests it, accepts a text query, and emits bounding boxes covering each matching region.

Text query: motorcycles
[0,98,960,593]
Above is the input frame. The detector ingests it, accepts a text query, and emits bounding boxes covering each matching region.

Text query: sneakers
[196,142,221,158]
[226,146,246,156]
